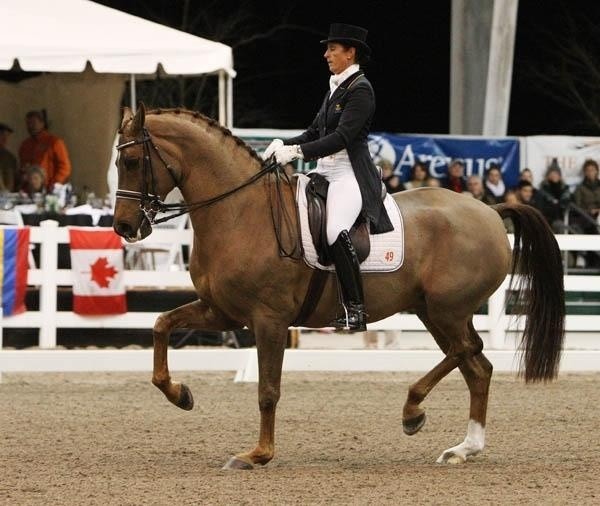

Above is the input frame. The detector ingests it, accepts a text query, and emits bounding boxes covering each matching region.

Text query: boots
[328,230,366,332]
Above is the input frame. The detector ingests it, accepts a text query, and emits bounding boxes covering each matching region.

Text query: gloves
[262,138,302,167]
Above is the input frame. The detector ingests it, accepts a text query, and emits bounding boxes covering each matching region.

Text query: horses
[112,99,567,471]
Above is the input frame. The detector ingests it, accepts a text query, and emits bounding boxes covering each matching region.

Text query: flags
[69,226,128,316]
[0,228,32,316]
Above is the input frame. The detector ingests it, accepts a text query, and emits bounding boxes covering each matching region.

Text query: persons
[374,157,598,311]
[1,110,72,218]
[262,21,395,336]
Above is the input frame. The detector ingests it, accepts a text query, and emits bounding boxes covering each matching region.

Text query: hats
[319,22,372,66]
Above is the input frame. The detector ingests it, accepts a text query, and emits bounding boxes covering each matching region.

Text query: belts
[322,153,348,163]
